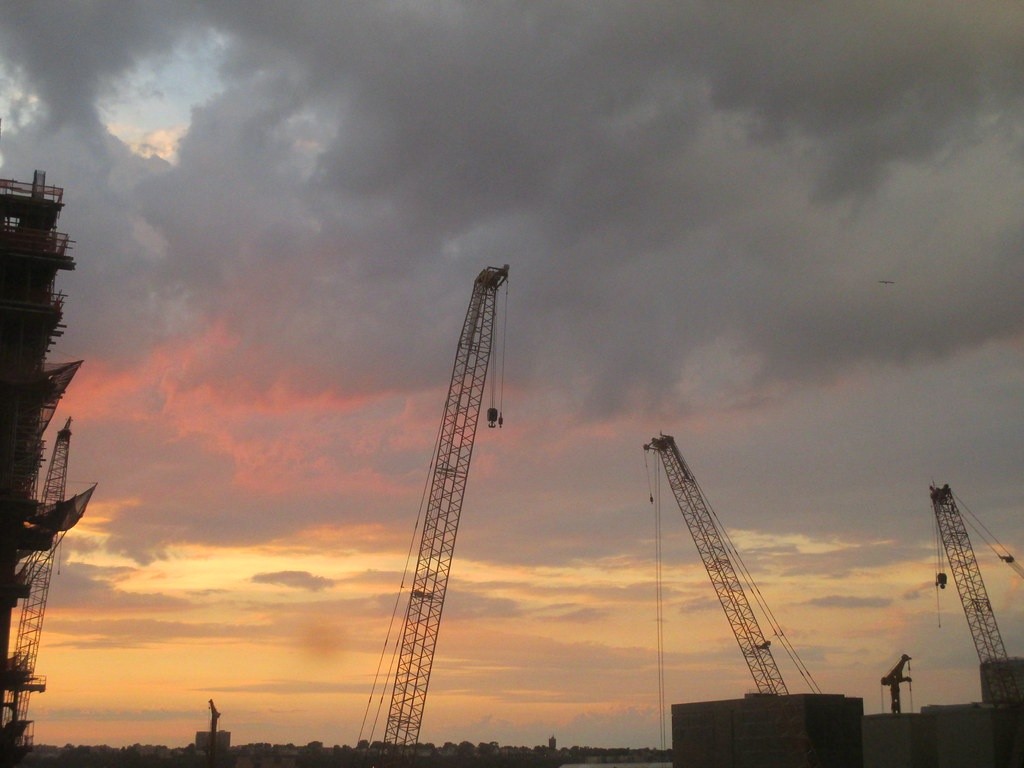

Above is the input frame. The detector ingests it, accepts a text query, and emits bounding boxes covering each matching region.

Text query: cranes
[881,654,914,713]
[358,263,512,768]
[642,428,822,767]
[926,482,1024,768]
[0,415,73,751]
